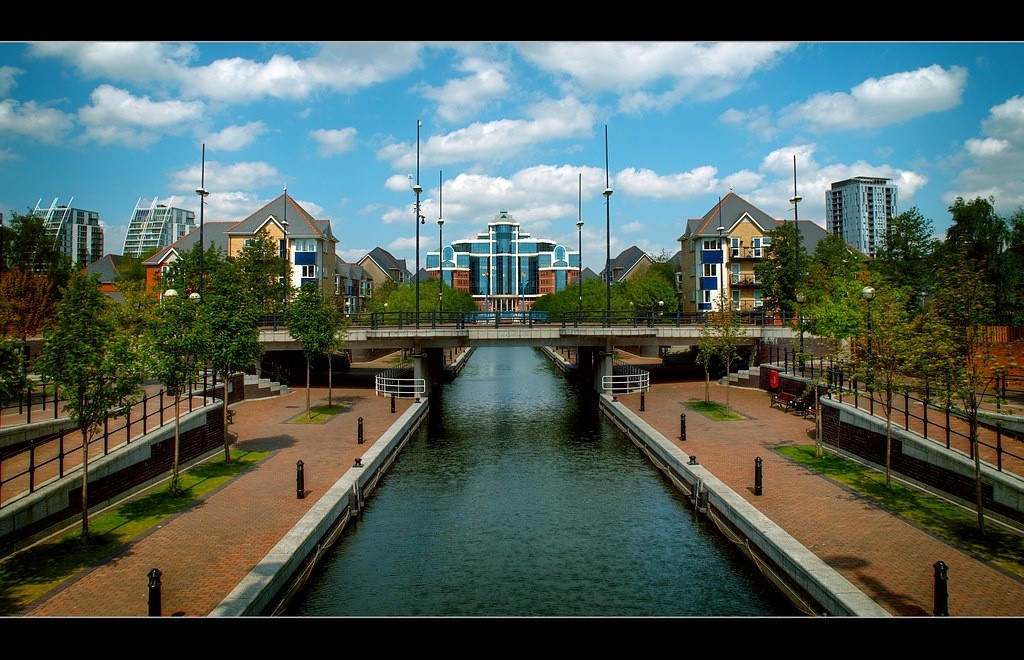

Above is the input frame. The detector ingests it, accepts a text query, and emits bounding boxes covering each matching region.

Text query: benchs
[805,403,817,418]
[771,391,797,413]
[226,410,237,424]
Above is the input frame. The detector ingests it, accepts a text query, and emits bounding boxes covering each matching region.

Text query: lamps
[147,568,162,587]
[296,460,305,471]
[680,413,686,421]
[358,417,364,424]
[754,458,762,467]
[933,561,950,579]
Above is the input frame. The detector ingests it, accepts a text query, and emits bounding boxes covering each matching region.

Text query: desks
[793,398,807,416]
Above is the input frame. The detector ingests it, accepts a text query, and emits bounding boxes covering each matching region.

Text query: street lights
[408,120,425,329]
[789,155,803,264]
[716,196,725,315]
[437,170,445,325]
[188,293,201,304]
[196,142,210,304]
[164,289,179,301]
[602,124,613,328]
[281,181,289,305]
[576,173,584,325]
[796,292,808,372]
[862,286,877,392]
